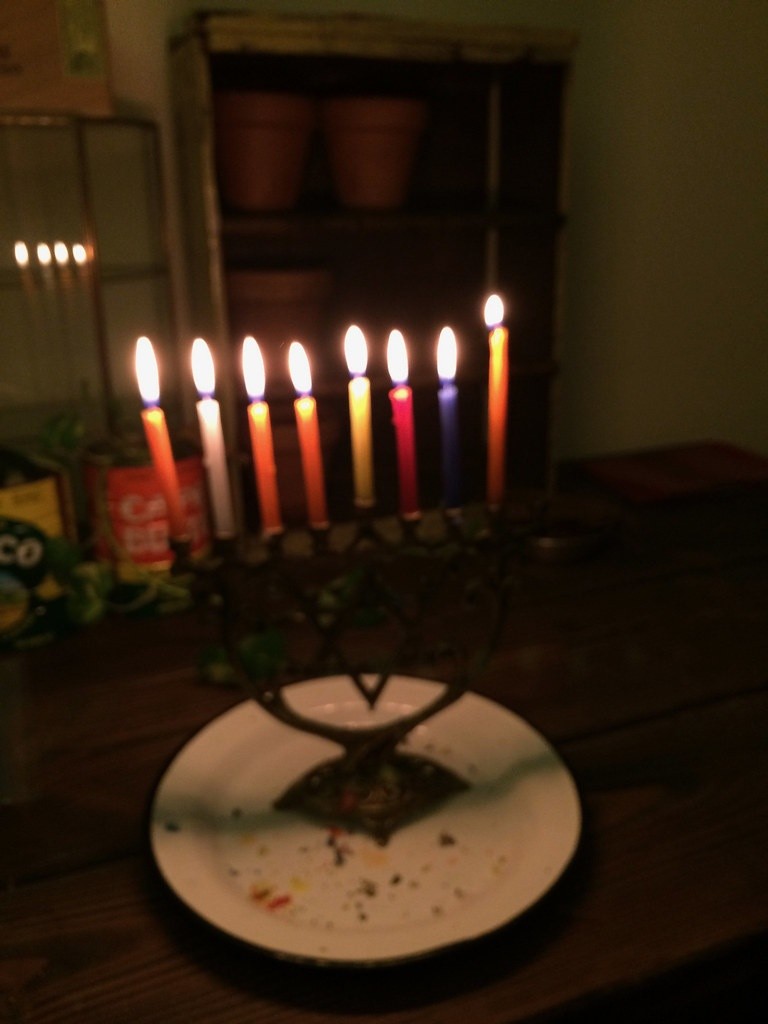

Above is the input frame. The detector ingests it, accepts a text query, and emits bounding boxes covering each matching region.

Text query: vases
[323,99,426,211]
[215,90,320,211]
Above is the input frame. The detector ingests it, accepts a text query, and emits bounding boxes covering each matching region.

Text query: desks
[0,440,768,1024]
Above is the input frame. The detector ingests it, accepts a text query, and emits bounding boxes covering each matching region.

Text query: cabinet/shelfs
[170,12,573,495]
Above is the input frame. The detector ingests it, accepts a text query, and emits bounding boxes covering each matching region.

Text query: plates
[151,672,581,961]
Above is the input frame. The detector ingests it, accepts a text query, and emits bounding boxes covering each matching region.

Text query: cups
[228,270,333,384]
[217,94,318,208]
[328,100,424,212]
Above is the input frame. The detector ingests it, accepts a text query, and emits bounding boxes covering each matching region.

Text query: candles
[195,398,233,534]
[246,400,281,528]
[295,396,328,524]
[388,386,420,513]
[488,325,508,504]
[348,376,375,506]
[141,407,186,534]
[436,386,460,494]
